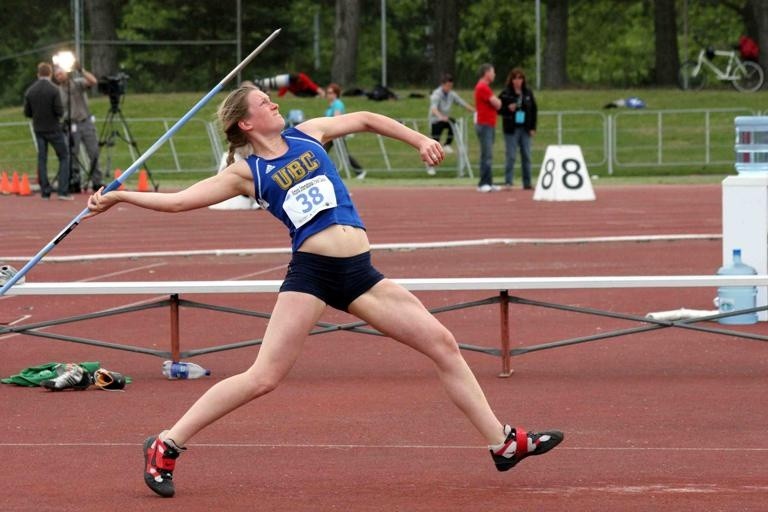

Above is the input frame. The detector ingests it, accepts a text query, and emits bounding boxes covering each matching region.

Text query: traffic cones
[9,172,20,194]
[113,169,126,190]
[136,168,154,192]
[18,173,32,196]
[0,172,11,196]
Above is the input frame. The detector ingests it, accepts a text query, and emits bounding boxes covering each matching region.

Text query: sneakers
[44,367,125,391]
[144,429,187,497]
[41,193,72,200]
[487,424,564,471]
[477,184,501,192]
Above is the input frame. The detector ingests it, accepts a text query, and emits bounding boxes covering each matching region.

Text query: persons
[81,81,564,498]
[474,63,502,192]
[426,74,476,175]
[52,62,107,195]
[496,69,537,190]
[322,84,366,180]
[24,63,74,201]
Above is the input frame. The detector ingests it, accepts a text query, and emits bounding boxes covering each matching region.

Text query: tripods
[50,72,101,189]
[84,108,159,191]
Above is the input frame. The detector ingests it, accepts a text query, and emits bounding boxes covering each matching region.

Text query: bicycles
[675,34,763,93]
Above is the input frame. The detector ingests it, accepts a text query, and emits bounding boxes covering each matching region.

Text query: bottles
[162,360,212,380]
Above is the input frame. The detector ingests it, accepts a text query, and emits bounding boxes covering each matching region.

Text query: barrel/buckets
[733,115,768,172]
[716,249,758,325]
